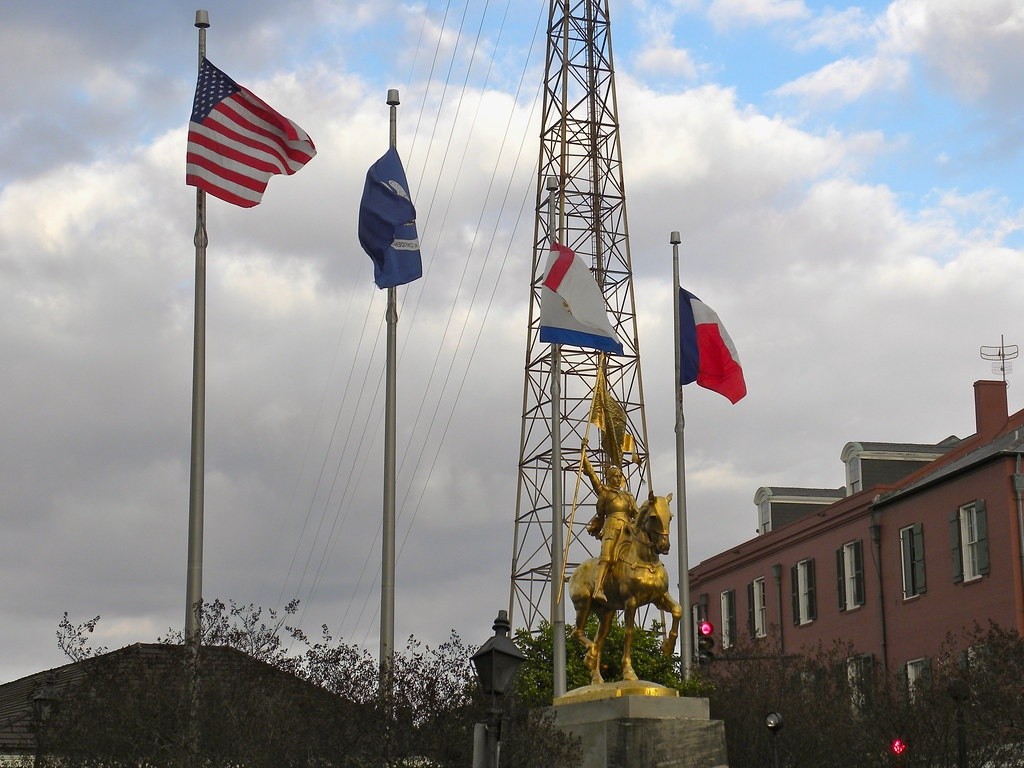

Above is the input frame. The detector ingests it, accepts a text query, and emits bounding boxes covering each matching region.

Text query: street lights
[467,609,525,767]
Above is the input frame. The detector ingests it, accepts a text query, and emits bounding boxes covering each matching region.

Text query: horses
[569,491,683,684]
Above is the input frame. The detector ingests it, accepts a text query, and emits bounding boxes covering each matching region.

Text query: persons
[580,437,640,605]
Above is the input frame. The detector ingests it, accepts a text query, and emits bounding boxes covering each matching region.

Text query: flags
[357,142,423,290]
[186,53,317,210]
[539,242,626,356]
[679,285,748,405]
[592,372,645,468]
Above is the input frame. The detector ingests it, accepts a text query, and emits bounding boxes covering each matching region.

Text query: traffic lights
[693,618,715,667]
